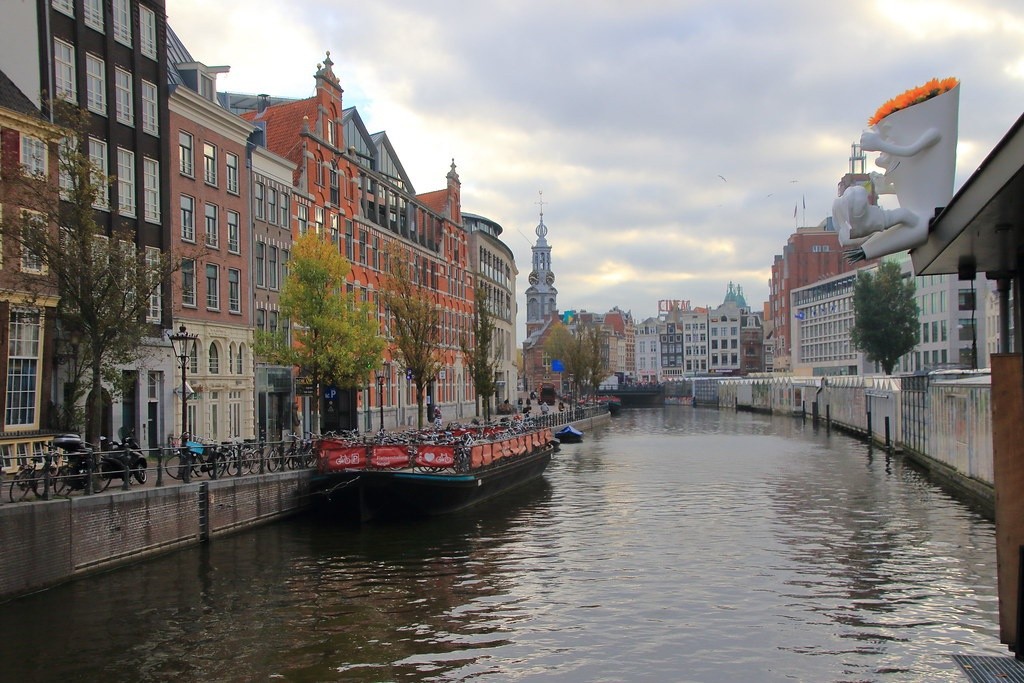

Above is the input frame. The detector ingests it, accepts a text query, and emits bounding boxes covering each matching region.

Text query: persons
[558,402,564,411]
[293,401,303,448]
[526,398,531,405]
[523,403,531,422]
[541,401,549,415]
[433,407,443,427]
[518,397,523,405]
[503,398,509,404]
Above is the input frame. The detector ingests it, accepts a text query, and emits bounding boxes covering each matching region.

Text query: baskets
[185,441,203,454]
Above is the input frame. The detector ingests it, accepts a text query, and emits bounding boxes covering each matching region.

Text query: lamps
[350,146,356,152]
[303,116,308,121]
[326,51,330,58]
[336,78,340,83]
[317,63,322,70]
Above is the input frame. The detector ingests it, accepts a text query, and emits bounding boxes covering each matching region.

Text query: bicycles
[10,425,453,502]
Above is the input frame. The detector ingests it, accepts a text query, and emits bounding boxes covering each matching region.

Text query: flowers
[867,75,958,127]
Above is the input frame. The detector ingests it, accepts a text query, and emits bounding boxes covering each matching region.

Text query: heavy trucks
[538,382,556,405]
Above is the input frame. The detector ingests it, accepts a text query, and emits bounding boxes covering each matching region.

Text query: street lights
[167,322,202,482]
[377,373,385,431]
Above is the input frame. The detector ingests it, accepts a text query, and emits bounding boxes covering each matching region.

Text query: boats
[599,394,621,412]
[555,424,583,443]
[310,421,562,512]
